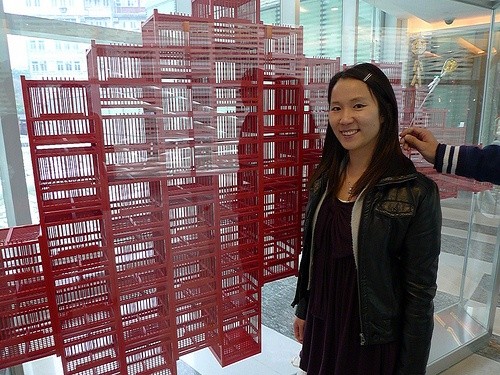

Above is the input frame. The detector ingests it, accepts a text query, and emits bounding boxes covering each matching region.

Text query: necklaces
[345,167,352,202]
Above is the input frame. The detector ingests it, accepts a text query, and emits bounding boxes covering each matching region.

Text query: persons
[399,127,500,186]
[236,68,317,274]
[291,63,442,375]
